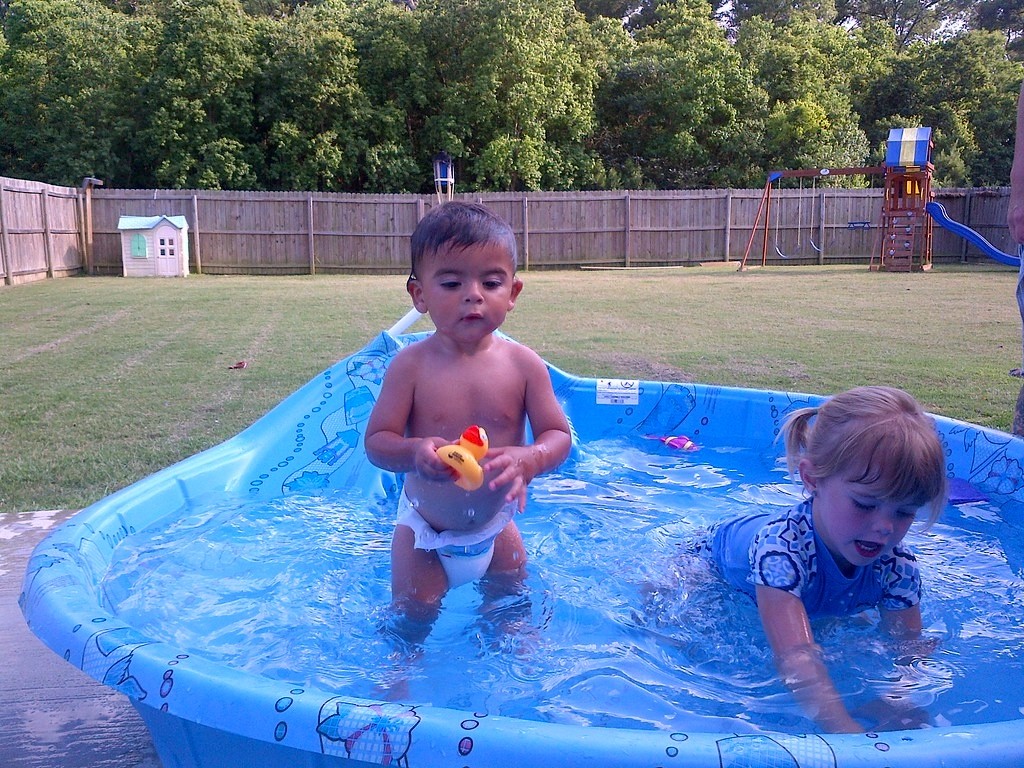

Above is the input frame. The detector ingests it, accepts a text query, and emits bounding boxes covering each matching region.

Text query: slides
[926,200,1020,268]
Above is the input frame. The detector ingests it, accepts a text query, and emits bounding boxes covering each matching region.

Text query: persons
[1006,84,1024,378]
[364,200,572,602]
[713,386,945,647]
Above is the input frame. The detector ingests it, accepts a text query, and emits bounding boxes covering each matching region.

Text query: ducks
[434,424,489,492]
